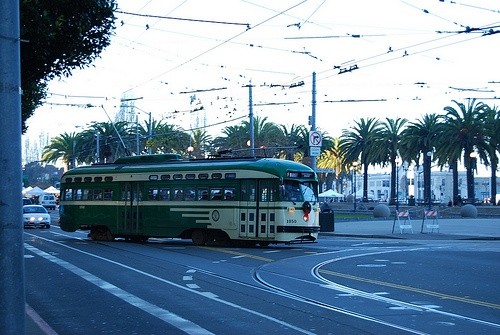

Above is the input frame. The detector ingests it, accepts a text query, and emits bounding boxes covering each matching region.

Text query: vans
[40,195,56,210]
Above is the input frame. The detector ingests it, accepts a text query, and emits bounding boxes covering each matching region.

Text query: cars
[22,198,32,205]
[23,205,51,228]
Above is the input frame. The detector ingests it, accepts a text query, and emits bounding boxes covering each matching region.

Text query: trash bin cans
[319,202,335,232]
[408,195,415,206]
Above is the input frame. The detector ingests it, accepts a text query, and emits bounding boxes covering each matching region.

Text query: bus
[57,145,321,248]
